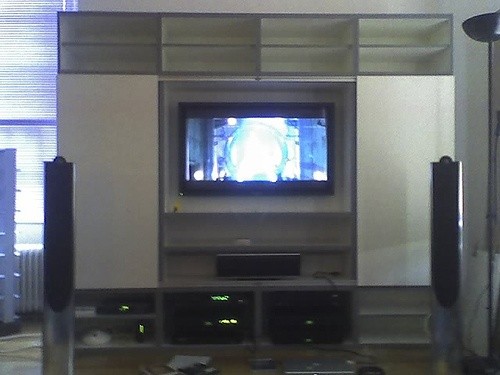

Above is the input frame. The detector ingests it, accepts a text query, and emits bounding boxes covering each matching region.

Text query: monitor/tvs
[177,102,334,198]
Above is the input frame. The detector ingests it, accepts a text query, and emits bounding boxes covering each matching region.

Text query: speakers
[427,156,463,307]
[41,155,78,315]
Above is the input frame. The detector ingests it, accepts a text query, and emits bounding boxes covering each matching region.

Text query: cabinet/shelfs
[57,10,454,77]
[66,274,438,349]
